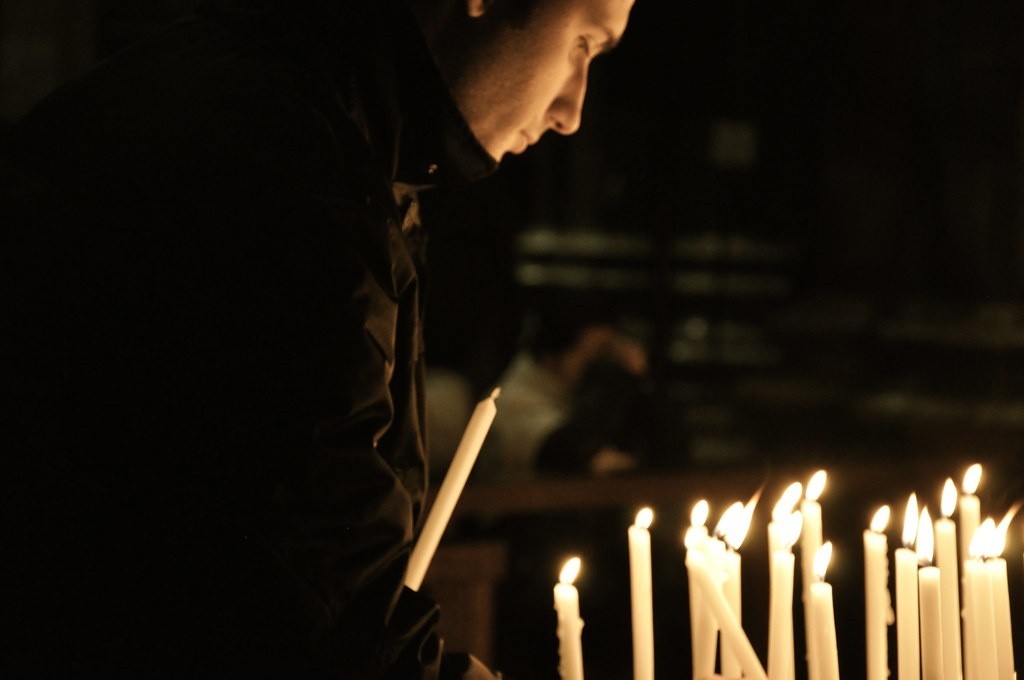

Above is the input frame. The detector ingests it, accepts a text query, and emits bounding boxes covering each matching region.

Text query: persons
[0,0,636,679]
[536,313,692,477]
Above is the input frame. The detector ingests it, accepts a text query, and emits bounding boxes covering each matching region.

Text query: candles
[403,385,502,591]
[546,462,1021,680]
